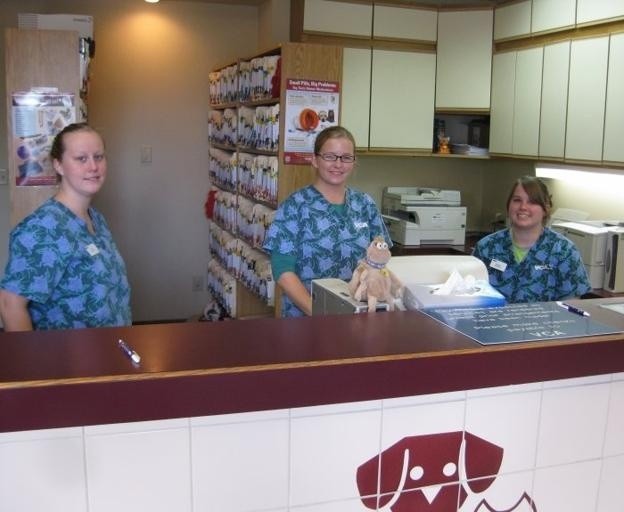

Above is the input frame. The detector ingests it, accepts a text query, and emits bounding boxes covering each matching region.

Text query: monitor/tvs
[357,253,505,313]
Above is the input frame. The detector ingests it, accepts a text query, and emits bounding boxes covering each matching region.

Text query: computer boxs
[306,276,390,316]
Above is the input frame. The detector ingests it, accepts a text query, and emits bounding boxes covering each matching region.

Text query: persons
[0,124,133,332]
[263,126,393,318]
[472,176,593,303]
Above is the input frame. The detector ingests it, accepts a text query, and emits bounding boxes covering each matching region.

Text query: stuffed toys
[349,236,402,314]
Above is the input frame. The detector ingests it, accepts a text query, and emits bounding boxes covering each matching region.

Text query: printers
[380,185,468,247]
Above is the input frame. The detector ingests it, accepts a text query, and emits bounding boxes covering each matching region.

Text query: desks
[389,236,623,297]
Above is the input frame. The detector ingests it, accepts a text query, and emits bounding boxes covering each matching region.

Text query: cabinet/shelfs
[2,29,96,265]
[208,40,343,323]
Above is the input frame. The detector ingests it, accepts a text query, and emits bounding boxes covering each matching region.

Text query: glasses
[315,152,357,164]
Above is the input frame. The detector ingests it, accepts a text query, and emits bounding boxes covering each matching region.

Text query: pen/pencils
[555,299,589,318]
[117,339,140,364]
